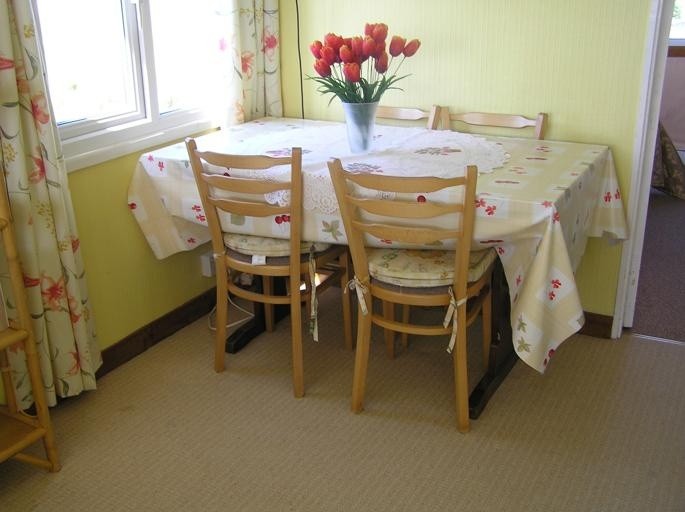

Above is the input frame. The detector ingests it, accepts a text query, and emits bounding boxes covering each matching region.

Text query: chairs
[326,153,498,432]
[396,104,548,346]
[262,104,440,347]
[186,137,355,400]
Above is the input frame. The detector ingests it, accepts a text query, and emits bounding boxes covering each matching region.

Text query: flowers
[303,21,423,150]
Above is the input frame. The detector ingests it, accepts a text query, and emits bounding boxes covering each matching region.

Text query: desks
[140,115,611,420]
